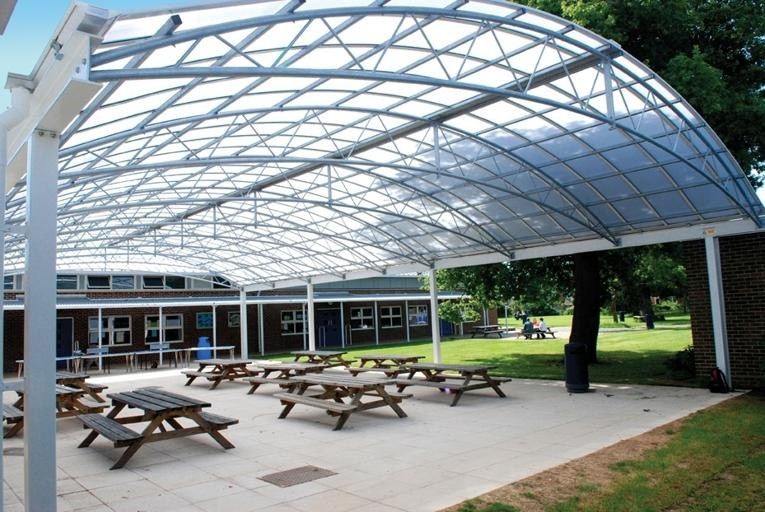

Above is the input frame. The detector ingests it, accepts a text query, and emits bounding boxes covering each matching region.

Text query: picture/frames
[228,311,240,328]
[195,311,213,330]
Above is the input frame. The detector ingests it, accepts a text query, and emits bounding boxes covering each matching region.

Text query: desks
[56,369,105,404]
[471,325,502,338]
[517,327,555,338]
[249,363,329,395]
[56,345,236,374]
[186,362,251,391]
[292,374,408,430]
[289,350,426,369]
[402,364,506,408]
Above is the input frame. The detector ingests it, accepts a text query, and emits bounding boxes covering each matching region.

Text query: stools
[15,360,24,379]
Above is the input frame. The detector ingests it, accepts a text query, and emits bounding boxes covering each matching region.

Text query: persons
[536,317,546,339]
[523,318,533,340]
[532,318,539,328]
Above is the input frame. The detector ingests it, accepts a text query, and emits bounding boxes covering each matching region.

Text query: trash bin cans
[197,337,211,360]
[565,343,588,393]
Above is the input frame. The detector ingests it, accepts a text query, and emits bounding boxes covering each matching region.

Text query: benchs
[437,374,510,383]
[272,394,356,414]
[185,372,228,380]
[394,379,465,393]
[469,330,503,338]
[367,390,412,401]
[233,369,264,374]
[3,382,238,471]
[517,332,556,339]
[248,378,295,386]
